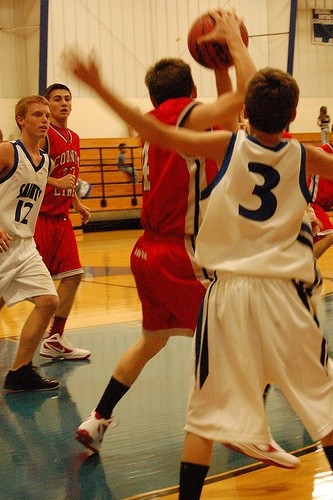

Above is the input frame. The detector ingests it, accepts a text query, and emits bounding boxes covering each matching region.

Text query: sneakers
[75,407,119,463]
[2,361,62,393]
[39,333,92,361]
[221,427,302,469]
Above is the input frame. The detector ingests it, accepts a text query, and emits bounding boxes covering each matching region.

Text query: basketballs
[187,8,249,69]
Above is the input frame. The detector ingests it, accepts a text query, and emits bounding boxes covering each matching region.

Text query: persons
[34,84,91,360]
[0,96,60,392]
[76,8,302,468]
[282,106,333,261]
[117,144,138,184]
[64,48,333,500]
